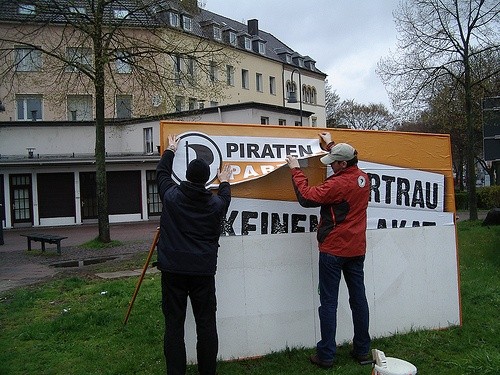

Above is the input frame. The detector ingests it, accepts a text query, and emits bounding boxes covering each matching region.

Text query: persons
[286,131,370,370]
[154,133,232,375]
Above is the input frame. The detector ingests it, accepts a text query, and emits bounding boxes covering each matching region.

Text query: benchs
[18,233,69,256]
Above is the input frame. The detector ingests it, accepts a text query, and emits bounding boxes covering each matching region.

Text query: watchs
[168,144,177,152]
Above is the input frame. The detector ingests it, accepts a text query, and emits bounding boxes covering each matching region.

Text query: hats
[320,143,356,165]
[186,158,210,183]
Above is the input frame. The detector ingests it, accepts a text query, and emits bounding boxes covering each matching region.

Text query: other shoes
[311,353,335,368]
[350,349,368,362]
